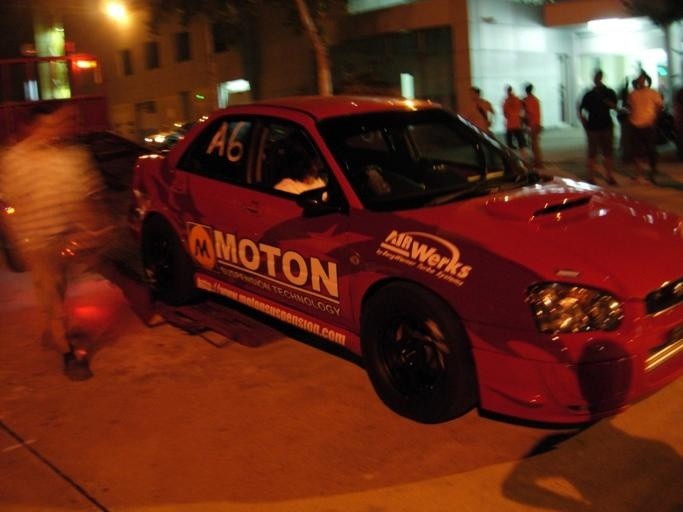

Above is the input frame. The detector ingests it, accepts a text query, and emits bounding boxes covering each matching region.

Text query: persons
[503,85,529,162]
[0,99,164,380]
[270,144,328,207]
[522,85,544,167]
[578,70,682,186]
[462,86,495,138]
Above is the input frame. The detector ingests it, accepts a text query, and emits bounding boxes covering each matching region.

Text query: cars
[127,95,682,424]
